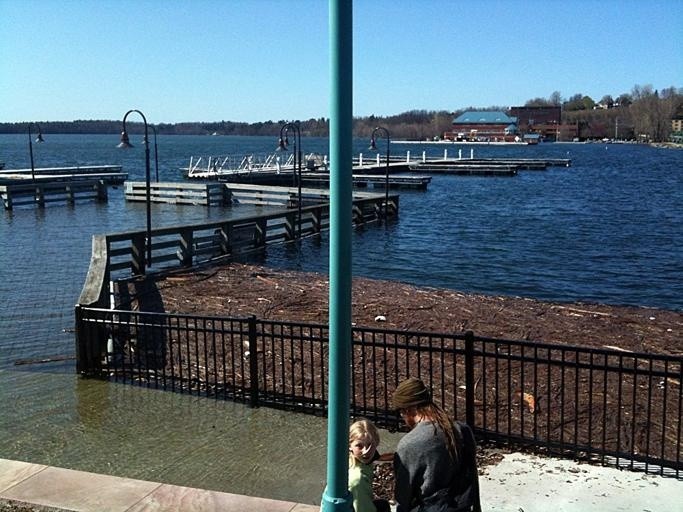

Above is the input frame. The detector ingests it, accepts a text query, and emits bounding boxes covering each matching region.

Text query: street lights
[275,123,301,239]
[28,122,45,200]
[116,109,152,268]
[141,124,159,197]
[368,126,389,226]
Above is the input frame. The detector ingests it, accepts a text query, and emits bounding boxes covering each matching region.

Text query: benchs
[287,189,385,210]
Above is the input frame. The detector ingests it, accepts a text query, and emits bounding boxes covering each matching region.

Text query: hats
[392,377,431,408]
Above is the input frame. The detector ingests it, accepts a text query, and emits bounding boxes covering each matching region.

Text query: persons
[389,376,482,512]
[348,417,390,511]
